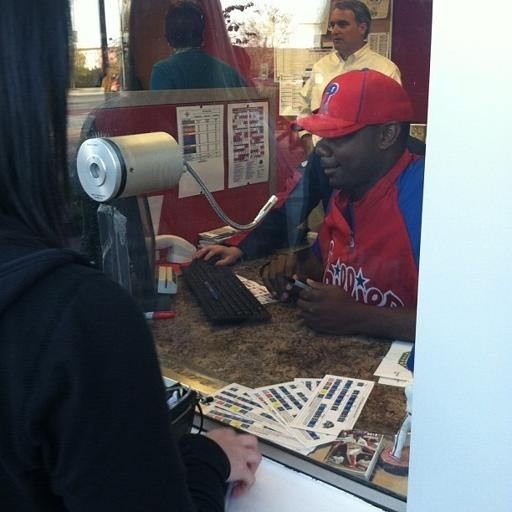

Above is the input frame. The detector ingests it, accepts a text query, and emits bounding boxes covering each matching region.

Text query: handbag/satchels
[163,382,204,437]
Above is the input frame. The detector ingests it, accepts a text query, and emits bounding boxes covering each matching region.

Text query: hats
[296,68,414,139]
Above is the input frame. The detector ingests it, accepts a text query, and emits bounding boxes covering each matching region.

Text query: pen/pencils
[142,311,176,319]
[283,276,309,290]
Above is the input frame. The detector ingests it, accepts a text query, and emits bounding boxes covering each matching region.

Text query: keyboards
[183,255,270,324]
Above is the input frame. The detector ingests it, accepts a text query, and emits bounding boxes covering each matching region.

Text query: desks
[138,232,408,512]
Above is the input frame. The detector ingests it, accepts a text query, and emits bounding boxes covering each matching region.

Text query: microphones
[186,156,277,231]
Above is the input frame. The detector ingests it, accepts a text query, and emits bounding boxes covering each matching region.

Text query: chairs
[293,137,425,245]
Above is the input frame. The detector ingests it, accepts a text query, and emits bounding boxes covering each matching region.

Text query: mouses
[204,250,226,261]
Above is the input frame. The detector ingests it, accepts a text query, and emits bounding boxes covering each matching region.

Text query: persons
[336,432,369,467]
[296,0,403,156]
[148,0,247,89]
[0,0,262,512]
[191,68,426,343]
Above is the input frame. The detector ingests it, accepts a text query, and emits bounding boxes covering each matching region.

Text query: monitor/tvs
[96,195,170,312]
